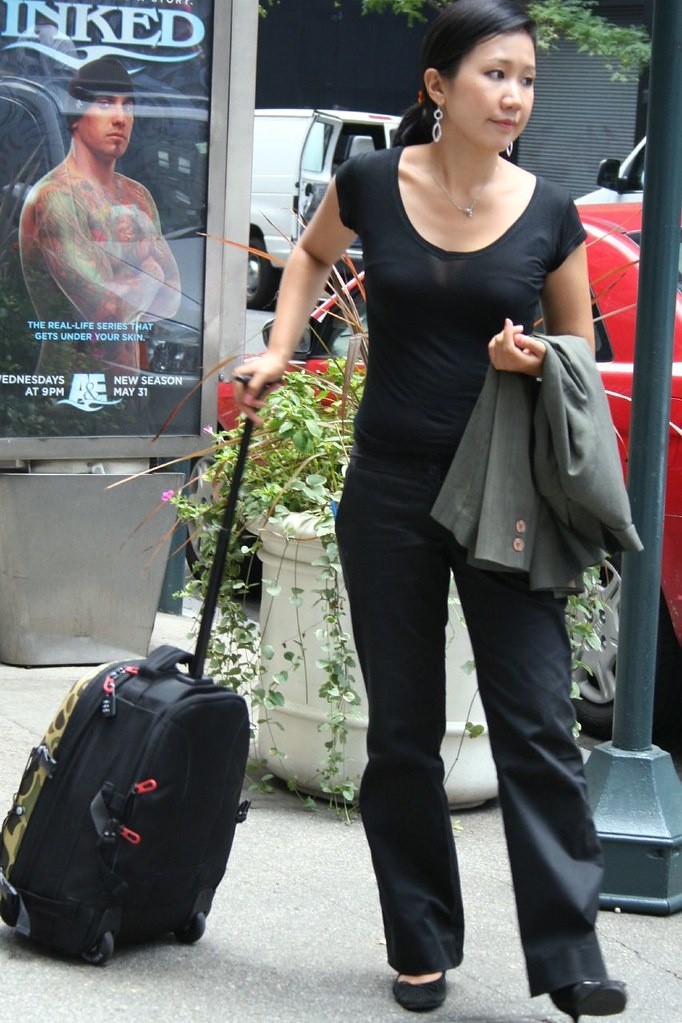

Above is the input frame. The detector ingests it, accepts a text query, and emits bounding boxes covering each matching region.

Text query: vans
[248,108,404,307]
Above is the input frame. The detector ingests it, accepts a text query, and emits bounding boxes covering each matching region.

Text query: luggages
[0,374,249,960]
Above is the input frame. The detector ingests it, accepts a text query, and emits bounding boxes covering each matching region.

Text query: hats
[62,54,134,131]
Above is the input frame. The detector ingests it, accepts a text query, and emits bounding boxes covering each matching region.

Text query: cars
[1,77,199,432]
[156,136,682,737]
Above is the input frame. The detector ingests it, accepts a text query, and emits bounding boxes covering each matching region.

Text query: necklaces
[424,158,500,218]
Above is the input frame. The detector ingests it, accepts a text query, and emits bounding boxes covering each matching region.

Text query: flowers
[104,206,612,824]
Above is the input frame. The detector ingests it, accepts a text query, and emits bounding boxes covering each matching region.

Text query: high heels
[550,979,626,1023]
[393,969,447,1009]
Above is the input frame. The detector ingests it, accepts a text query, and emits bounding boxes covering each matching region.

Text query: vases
[244,501,500,812]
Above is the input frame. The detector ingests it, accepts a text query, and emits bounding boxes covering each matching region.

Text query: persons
[231,0,628,1022]
[19,58,181,396]
